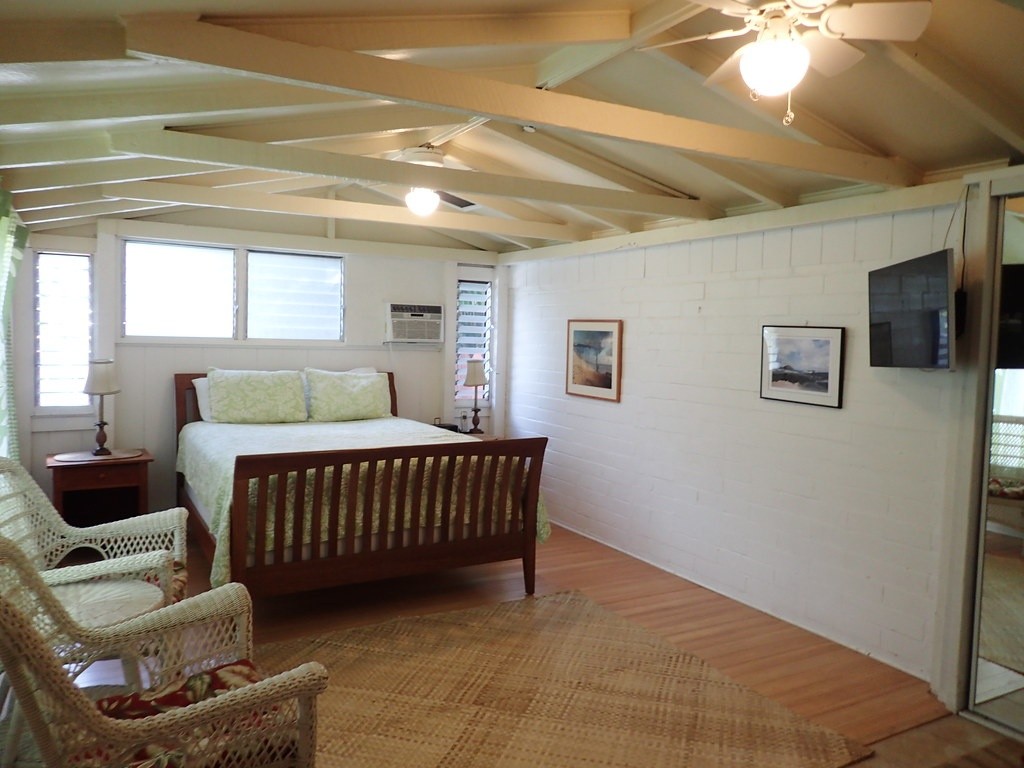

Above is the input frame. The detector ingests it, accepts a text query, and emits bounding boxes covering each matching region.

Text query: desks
[41,576,169,690]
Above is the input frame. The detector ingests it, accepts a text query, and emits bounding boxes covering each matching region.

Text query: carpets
[245,588,876,766]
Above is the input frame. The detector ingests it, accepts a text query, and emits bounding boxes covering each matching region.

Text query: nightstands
[466,433,503,442]
[44,449,156,565]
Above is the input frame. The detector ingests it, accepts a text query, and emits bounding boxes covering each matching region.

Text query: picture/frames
[564,318,622,402]
[758,325,845,409]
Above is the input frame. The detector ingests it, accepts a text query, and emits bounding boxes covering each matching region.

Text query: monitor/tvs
[869,248,956,370]
[996,264,1024,369]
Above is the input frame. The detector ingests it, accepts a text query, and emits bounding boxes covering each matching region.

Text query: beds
[169,414,551,597]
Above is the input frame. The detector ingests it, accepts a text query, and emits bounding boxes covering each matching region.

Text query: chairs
[0,534,328,767]
[1,457,188,621]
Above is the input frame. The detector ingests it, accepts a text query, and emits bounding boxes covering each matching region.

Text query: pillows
[190,376,208,422]
[304,367,394,422]
[301,366,377,414]
[207,366,308,422]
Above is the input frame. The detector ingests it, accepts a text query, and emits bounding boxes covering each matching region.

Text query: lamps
[400,146,448,217]
[82,359,122,456]
[741,19,812,101]
[462,358,490,434]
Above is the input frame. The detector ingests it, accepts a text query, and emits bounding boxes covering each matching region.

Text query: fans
[632,1,934,97]
